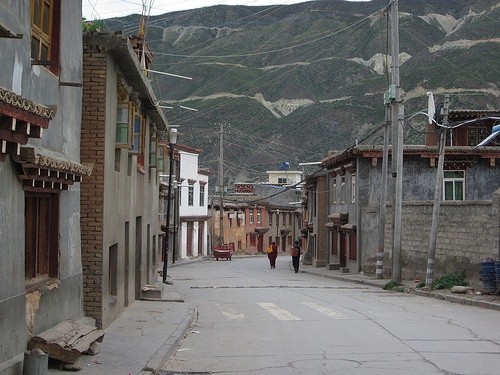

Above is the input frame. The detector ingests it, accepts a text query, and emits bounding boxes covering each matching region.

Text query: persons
[290,241,301,273]
[267,242,278,269]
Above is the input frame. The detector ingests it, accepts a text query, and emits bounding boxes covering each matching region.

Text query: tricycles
[214,244,232,261]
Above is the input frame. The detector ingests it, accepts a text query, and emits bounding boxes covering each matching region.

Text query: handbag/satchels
[290,248,298,257]
[267,244,273,253]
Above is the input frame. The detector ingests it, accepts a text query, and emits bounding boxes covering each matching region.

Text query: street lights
[162,128,178,284]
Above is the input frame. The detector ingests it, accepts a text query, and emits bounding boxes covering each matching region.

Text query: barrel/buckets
[22,350,49,375]
